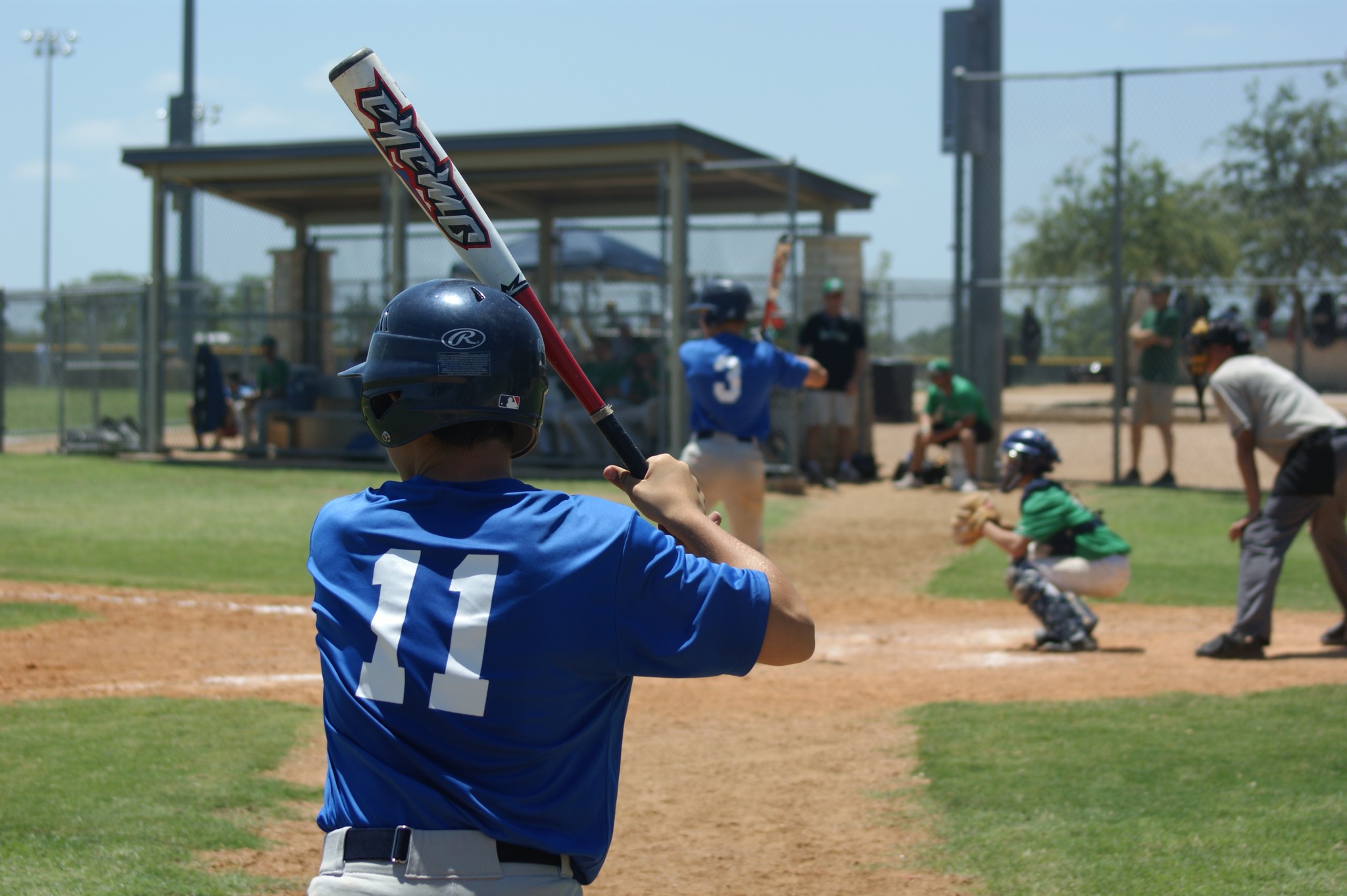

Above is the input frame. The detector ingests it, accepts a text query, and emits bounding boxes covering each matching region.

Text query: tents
[448,228,694,330]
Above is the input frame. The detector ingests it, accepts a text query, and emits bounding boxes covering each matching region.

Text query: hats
[1185,320,1240,345]
[824,279,843,293]
[926,358,949,373]
[258,335,275,346]
[1153,282,1170,294]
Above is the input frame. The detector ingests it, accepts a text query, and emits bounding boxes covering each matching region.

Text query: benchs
[254,376,365,453]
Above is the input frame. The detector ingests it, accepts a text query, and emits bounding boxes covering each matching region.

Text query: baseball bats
[326,47,652,482]
[761,236,794,335]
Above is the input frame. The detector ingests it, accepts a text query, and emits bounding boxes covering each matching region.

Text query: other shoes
[1037,633,1094,649]
[1152,475,1173,486]
[962,480,977,490]
[1124,473,1138,483]
[1195,633,1263,659]
[1323,623,1347,642]
[893,479,916,488]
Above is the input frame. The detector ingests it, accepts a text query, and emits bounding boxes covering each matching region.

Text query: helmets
[1002,428,1052,460]
[337,279,548,459]
[687,279,750,316]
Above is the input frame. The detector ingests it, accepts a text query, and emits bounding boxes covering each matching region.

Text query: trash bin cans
[872,360,915,421]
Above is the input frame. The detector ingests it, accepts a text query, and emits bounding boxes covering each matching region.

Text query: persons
[188,334,317,460]
[799,276,869,485]
[891,355,997,495]
[676,277,828,552]
[1194,319,1347,659]
[1112,283,1192,490]
[306,279,815,896]
[952,429,1133,653]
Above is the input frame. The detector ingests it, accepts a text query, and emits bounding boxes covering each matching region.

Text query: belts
[342,824,578,869]
[695,425,754,443]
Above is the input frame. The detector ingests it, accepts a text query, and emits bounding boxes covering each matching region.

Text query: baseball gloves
[948,496,1002,545]
